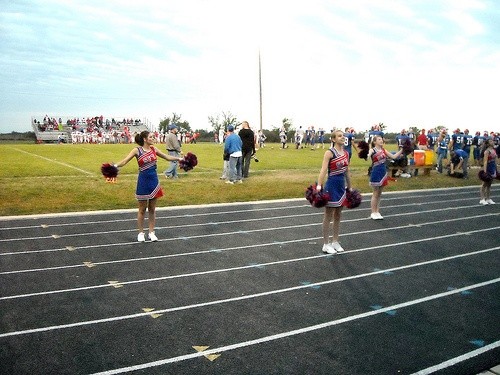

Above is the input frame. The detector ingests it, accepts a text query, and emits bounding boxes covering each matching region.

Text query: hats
[169,124,177,129]
[228,125,234,131]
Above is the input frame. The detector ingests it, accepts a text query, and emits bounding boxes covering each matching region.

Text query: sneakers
[486,199,495,205]
[137,232,145,242]
[377,213,383,220]
[371,213,378,220]
[332,242,344,252]
[480,200,488,205]
[148,231,158,242]
[322,243,337,254]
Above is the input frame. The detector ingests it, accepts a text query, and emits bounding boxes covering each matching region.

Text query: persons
[473,131,500,167]
[437,127,473,180]
[342,127,358,163]
[479,140,498,205]
[176,130,200,144]
[388,128,436,177]
[364,130,370,143]
[112,130,183,243]
[34,114,141,145]
[165,125,182,179]
[214,121,267,185]
[366,135,403,220]
[278,125,325,150]
[368,125,384,147]
[316,129,352,253]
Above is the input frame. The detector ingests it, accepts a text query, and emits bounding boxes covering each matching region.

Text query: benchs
[387,165,433,177]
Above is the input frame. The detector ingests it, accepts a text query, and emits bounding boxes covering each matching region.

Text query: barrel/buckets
[414,149,425,166]
[425,150,436,166]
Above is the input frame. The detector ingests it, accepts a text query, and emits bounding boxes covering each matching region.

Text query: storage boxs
[414,150,425,166]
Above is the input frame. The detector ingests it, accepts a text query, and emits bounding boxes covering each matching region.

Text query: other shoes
[234,179,242,184]
[225,179,234,185]
